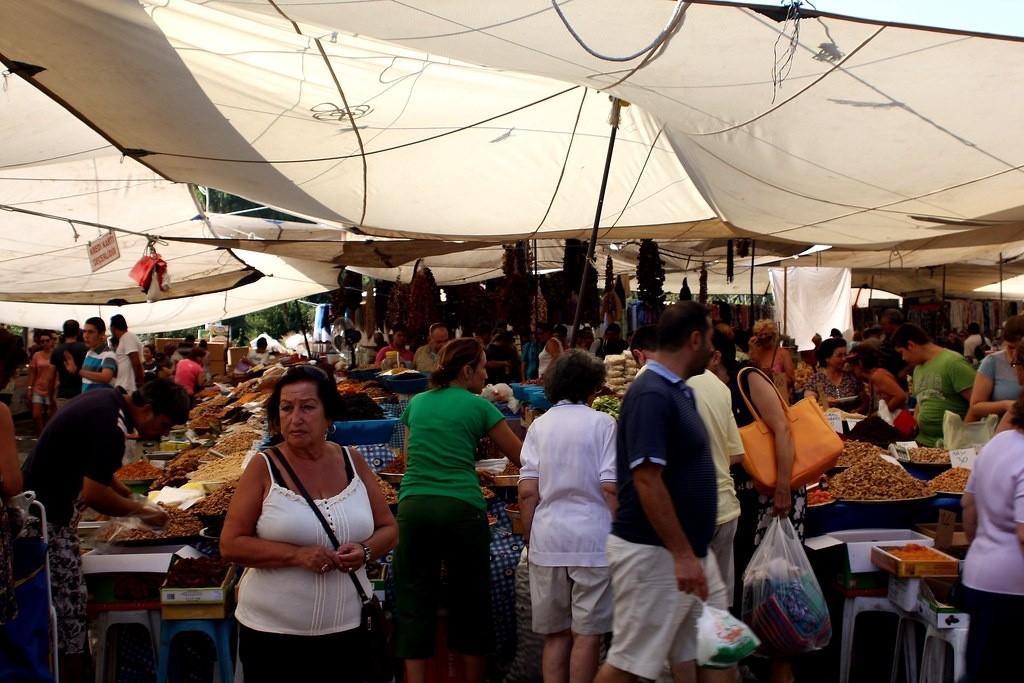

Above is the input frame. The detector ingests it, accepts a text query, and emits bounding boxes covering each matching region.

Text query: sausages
[163,554,229,588]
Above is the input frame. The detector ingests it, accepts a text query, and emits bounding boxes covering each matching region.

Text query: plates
[836,396,858,402]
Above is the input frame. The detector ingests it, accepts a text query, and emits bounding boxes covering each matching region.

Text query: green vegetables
[591,394,622,419]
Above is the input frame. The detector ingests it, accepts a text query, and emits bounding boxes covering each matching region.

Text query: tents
[1,0,1024,336]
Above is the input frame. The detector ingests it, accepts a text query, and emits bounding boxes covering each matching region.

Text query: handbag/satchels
[361,593,396,683]
[889,407,918,441]
[740,513,834,659]
[729,366,844,496]
[942,410,998,451]
[974,333,991,362]
[831,351,847,359]
[693,594,762,670]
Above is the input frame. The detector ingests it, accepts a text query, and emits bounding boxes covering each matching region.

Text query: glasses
[52,338,58,341]
[40,338,52,343]
[757,332,775,343]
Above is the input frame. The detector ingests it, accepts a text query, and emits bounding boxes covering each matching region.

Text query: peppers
[332,237,753,339]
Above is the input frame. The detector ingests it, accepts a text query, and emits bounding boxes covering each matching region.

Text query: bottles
[381,350,407,372]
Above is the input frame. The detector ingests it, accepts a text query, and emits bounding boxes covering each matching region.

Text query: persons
[809,308,1024,447]
[254,337,269,359]
[0,315,210,683]
[220,366,399,683]
[961,387,1024,683]
[320,322,415,376]
[395,300,796,683]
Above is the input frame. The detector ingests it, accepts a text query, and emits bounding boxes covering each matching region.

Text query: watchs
[360,543,372,565]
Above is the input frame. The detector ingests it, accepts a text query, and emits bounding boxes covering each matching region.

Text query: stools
[94,611,159,683]
[158,619,235,683]
[840,596,918,683]
[920,623,972,683]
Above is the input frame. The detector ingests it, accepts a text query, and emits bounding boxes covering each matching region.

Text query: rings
[349,567,353,573]
[321,564,328,573]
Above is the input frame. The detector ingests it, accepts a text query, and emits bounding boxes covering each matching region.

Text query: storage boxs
[804,522,980,627]
[81,545,233,619]
[155,337,248,384]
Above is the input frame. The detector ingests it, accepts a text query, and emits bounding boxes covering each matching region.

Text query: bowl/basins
[509,381,551,410]
[346,369,432,393]
